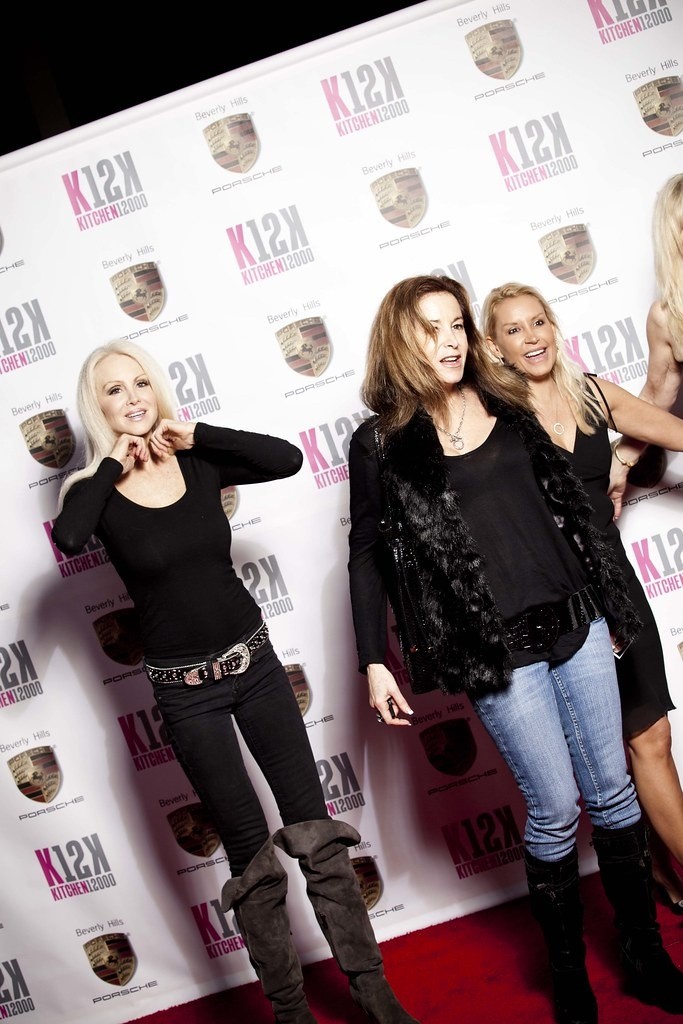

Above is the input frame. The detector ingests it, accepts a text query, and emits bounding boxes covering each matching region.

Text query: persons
[477,281,683,912]
[347,274,683,1024]
[605,172,683,521]
[51,342,421,1024]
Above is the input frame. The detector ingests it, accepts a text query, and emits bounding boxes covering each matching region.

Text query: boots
[522,841,598,1024]
[592,810,683,1013]
[221,833,317,1024]
[273,819,419,1024]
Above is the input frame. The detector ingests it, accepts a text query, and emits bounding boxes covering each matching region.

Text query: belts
[142,622,269,686]
[503,582,604,654]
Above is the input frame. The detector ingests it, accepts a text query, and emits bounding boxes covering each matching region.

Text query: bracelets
[613,444,639,467]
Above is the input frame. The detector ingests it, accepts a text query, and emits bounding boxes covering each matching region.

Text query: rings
[376,713,383,723]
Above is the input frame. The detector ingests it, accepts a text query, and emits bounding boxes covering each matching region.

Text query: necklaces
[434,386,466,451]
[540,398,565,435]
